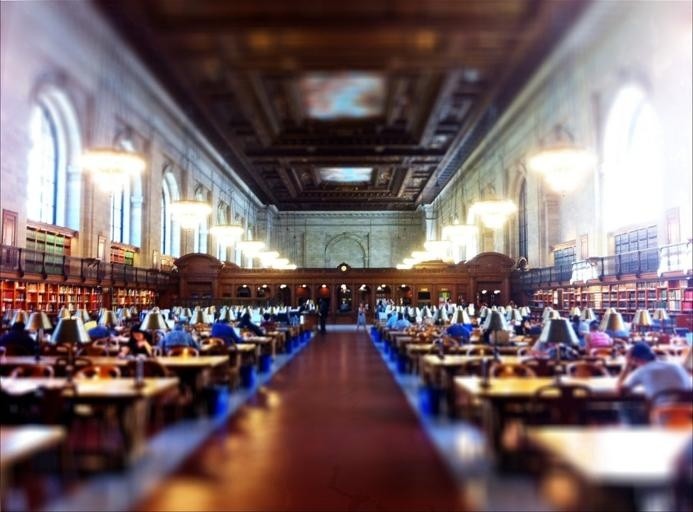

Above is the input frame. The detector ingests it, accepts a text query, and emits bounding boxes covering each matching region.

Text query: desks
[0,426,67,512]
[371,319,420,374]
[525,428,693,512]
[418,354,680,424]
[0,376,180,468]
[405,344,689,377]
[44,344,257,393]
[242,336,273,374]
[266,319,313,354]
[0,355,230,420]
[454,377,646,476]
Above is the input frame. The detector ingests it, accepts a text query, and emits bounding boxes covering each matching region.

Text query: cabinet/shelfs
[549,239,576,280]
[26,226,71,275]
[111,246,134,280]
[614,220,658,271]
[0,279,161,317]
[518,270,693,331]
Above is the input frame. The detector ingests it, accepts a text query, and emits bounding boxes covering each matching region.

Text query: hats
[631,342,656,361]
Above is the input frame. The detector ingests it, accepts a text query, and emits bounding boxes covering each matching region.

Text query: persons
[393,313,414,330]
[356,302,368,333]
[572,314,587,339]
[237,314,264,336]
[119,324,153,358]
[261,311,277,328]
[385,313,398,331]
[586,321,614,347]
[446,297,454,310]
[318,297,328,336]
[1,322,36,355]
[160,323,200,351]
[617,342,692,401]
[212,319,244,346]
[521,318,535,335]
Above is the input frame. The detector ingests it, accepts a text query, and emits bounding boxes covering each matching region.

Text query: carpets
[122,331,473,511]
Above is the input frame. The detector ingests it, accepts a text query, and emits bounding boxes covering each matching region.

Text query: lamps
[424,240,451,254]
[441,224,480,246]
[99,311,118,326]
[238,240,265,255]
[433,308,449,321]
[24,312,52,361]
[50,318,92,388]
[118,308,132,328]
[506,309,523,321]
[604,312,625,361]
[632,310,654,336]
[450,310,470,324]
[9,311,28,326]
[212,224,244,245]
[419,308,432,318]
[74,148,146,191]
[172,306,289,326]
[653,308,671,333]
[482,311,509,363]
[580,308,597,333]
[525,145,598,193]
[540,318,580,388]
[75,309,89,322]
[408,307,421,317]
[167,200,213,226]
[469,198,518,229]
[139,313,168,360]
